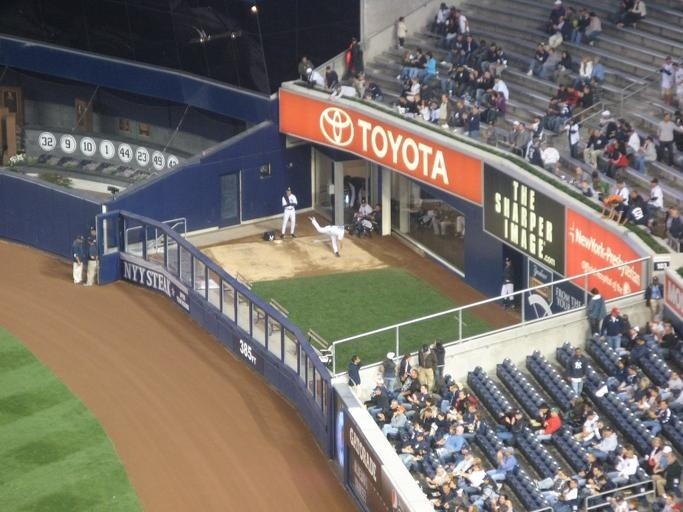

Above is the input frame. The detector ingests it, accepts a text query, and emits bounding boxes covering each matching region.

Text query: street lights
[198,33,241,85]
[250,5,270,92]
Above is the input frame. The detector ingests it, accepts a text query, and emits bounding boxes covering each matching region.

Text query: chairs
[389,329,683,512]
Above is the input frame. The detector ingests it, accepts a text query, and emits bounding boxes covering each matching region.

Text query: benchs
[362,0,682,220]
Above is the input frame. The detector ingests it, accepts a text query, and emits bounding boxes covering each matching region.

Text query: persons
[71,234,84,284]
[409,198,465,239]
[500,257,518,309]
[348,276,683,512]
[509,0,683,253]
[90,226,96,245]
[299,1,509,148]
[82,237,97,287]
[281,186,298,239]
[307,175,381,256]
